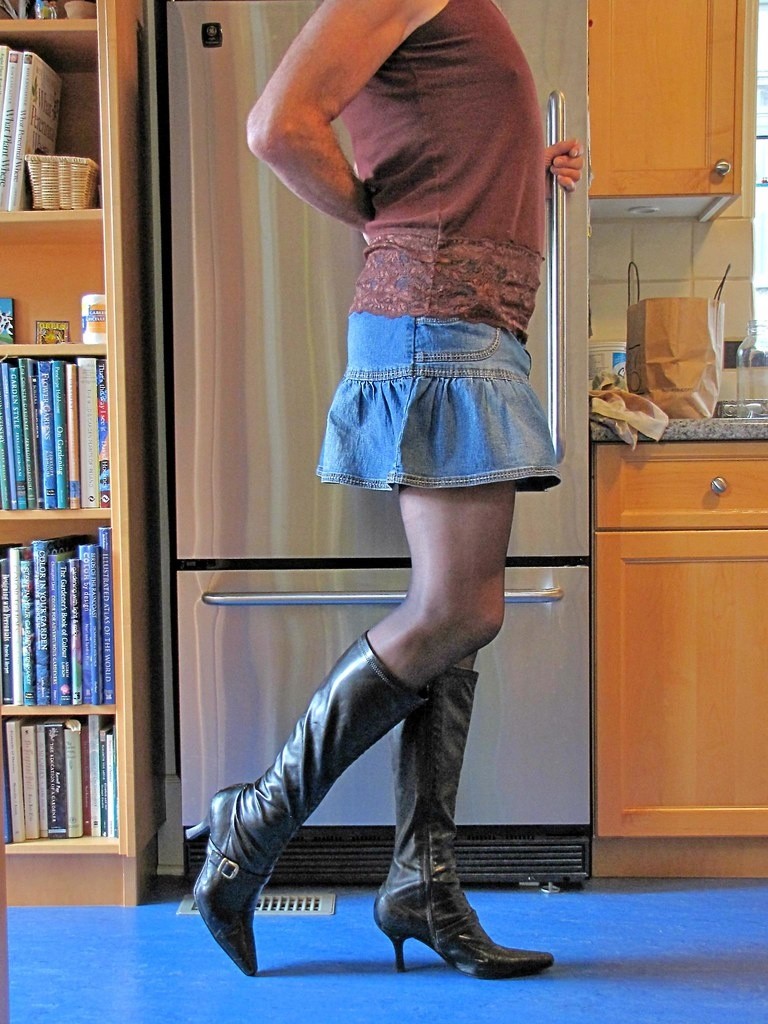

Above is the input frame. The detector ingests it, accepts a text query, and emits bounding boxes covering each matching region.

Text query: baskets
[23,154,99,209]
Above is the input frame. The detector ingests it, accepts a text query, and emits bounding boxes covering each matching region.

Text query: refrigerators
[146,0,590,893]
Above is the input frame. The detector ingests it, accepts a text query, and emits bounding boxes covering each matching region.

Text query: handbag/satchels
[626,262,732,419]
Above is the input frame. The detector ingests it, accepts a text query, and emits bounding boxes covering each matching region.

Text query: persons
[180,0,589,983]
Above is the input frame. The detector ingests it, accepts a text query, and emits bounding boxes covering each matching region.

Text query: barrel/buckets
[589,342,627,392]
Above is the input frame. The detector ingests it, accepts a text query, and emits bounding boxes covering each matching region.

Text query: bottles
[736,320,768,418]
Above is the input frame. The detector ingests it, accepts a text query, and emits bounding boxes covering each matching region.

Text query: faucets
[722,333,768,418]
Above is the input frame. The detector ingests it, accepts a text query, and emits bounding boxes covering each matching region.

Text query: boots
[186,630,429,976]
[374,666,555,979]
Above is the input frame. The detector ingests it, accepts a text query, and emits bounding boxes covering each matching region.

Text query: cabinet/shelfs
[0,0,166,908]
[587,0,744,221]
[590,440,768,877]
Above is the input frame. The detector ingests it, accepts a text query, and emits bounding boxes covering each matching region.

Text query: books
[0,44,64,213]
[0,356,119,841]
